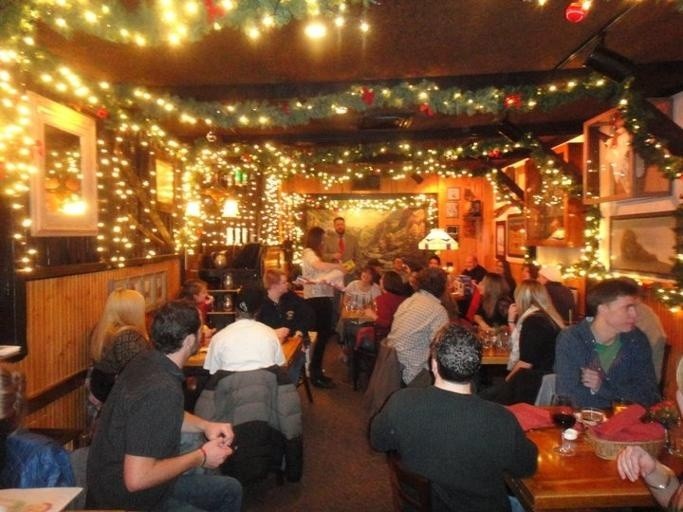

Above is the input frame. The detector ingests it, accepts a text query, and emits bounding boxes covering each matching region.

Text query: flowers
[651,400,679,430]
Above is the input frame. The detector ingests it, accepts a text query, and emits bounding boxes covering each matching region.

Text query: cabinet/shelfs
[582,99,673,205]
[524,142,583,249]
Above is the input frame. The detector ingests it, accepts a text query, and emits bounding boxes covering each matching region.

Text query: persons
[429,254,440,266]
[256,269,308,341]
[388,266,449,386]
[198,290,289,396]
[0,354,76,488]
[371,323,538,512]
[180,278,214,327]
[85,288,222,476]
[478,278,564,407]
[555,278,665,410]
[475,293,515,333]
[461,256,488,285]
[617,356,683,511]
[364,259,385,286]
[303,226,358,388]
[467,273,518,333]
[357,271,410,342]
[497,259,517,294]
[345,266,382,310]
[84,301,243,512]
[323,217,365,288]
[394,258,411,282]
[523,263,538,280]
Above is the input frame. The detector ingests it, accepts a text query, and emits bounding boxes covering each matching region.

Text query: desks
[0,486,85,511]
[150,336,303,373]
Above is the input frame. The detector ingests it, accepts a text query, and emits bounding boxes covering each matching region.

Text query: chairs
[297,362,313,403]
[198,243,264,283]
[385,448,431,512]
[353,326,390,391]
[634,303,667,385]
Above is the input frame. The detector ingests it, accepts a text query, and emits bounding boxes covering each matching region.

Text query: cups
[612,400,632,415]
[223,272,233,288]
[557,429,580,441]
[478,330,511,354]
[346,303,370,316]
[222,293,234,311]
[667,417,683,457]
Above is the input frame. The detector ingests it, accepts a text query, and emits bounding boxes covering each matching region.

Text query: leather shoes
[309,367,330,384]
[309,377,333,389]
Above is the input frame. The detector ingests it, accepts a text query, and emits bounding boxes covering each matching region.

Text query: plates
[572,411,608,426]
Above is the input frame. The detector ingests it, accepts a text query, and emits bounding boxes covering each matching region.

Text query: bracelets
[644,465,674,489]
[199,448,206,468]
[641,457,656,479]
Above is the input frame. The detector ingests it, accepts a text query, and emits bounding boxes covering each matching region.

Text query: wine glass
[550,399,576,454]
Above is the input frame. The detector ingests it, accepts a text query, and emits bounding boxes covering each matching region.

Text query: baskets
[592,427,669,460]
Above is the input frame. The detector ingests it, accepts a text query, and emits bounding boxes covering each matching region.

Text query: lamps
[417,228,459,251]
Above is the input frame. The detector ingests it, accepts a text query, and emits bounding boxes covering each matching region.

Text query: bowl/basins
[583,428,668,460]
[582,407,603,445]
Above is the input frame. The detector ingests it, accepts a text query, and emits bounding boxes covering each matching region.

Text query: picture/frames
[25,90,99,238]
[508,213,526,259]
[147,147,177,215]
[610,210,683,277]
[445,225,460,250]
[447,187,460,201]
[495,221,506,259]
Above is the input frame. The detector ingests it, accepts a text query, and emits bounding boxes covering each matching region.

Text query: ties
[339,236,346,254]
